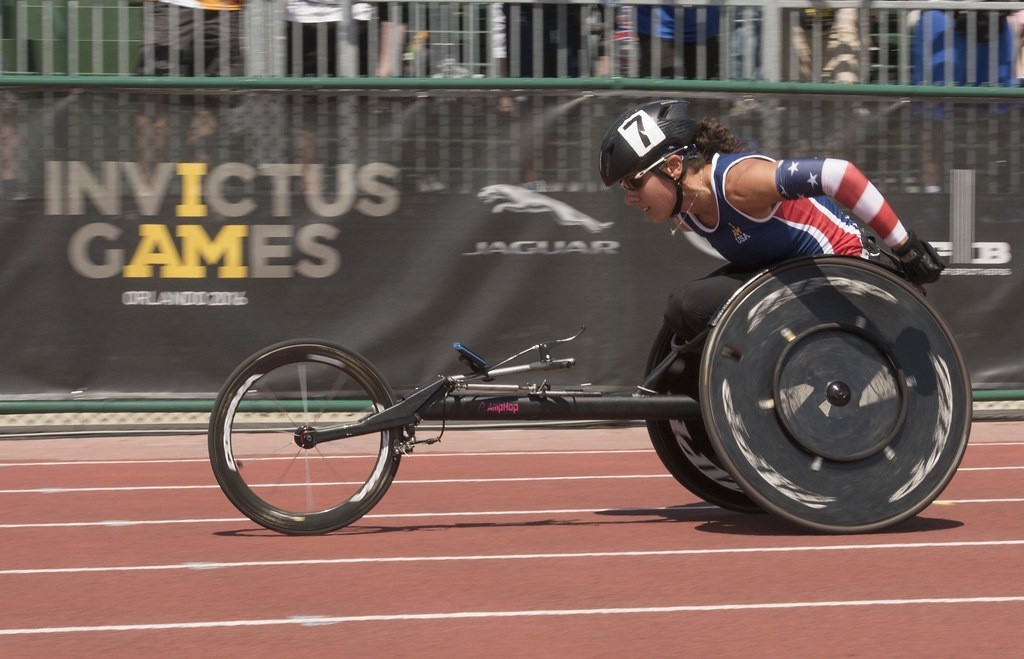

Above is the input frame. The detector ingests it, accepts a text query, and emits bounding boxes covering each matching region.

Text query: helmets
[598,99,698,187]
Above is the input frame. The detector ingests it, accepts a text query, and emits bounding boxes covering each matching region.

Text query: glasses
[619,145,692,192]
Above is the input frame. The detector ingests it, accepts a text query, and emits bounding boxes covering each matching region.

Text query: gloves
[891,230,946,286]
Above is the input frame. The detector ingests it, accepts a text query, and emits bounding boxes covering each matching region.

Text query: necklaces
[668,163,703,236]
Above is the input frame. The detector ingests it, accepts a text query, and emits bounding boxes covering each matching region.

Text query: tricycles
[207,247,971,537]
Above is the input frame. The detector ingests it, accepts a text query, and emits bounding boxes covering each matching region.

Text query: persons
[598,98,943,356]
